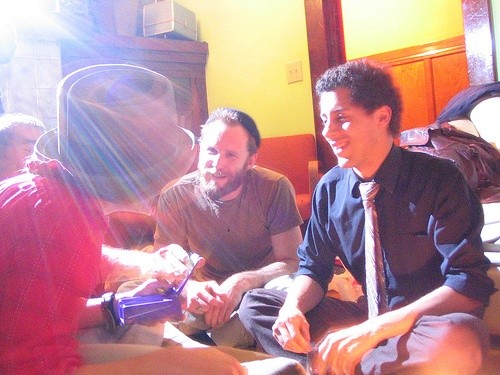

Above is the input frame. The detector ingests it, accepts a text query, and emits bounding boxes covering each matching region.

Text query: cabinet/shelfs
[60,36,208,176]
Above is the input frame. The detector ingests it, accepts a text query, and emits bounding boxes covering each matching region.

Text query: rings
[197,306,202,310]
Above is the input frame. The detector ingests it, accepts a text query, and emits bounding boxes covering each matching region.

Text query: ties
[359,182,388,320]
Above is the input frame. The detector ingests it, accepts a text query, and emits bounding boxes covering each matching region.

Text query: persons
[152,105,304,348]
[0,113,205,300]
[0,64,248,375]
[237,60,500,375]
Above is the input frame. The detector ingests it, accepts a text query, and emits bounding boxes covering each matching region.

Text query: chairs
[256,133,325,237]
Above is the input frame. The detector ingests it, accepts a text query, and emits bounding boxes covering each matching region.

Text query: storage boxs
[143,0,197,41]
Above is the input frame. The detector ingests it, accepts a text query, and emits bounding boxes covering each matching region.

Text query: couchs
[402,91,500,345]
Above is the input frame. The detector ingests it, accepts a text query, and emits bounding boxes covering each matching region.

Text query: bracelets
[101,292,121,331]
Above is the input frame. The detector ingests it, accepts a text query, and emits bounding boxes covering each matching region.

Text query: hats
[33,64,197,205]
